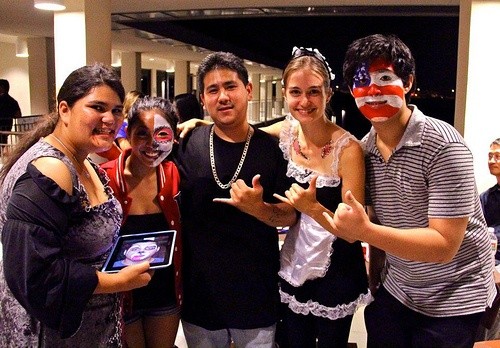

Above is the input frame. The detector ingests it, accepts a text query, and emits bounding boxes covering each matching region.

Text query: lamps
[111,51,121,68]
[34,0,66,11]
[15,40,28,57]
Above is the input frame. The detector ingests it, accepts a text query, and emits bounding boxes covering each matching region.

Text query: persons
[1,62,155,347]
[96,96,185,347]
[473,138,500,341]
[112,91,204,152]
[175,46,375,348]
[156,51,301,347]
[0,79,22,157]
[113,238,166,268]
[322,33,500,348]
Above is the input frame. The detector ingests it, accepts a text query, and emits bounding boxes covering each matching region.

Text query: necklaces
[209,122,253,189]
[49,131,91,180]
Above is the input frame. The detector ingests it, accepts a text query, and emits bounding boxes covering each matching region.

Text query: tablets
[100,230,177,274]
